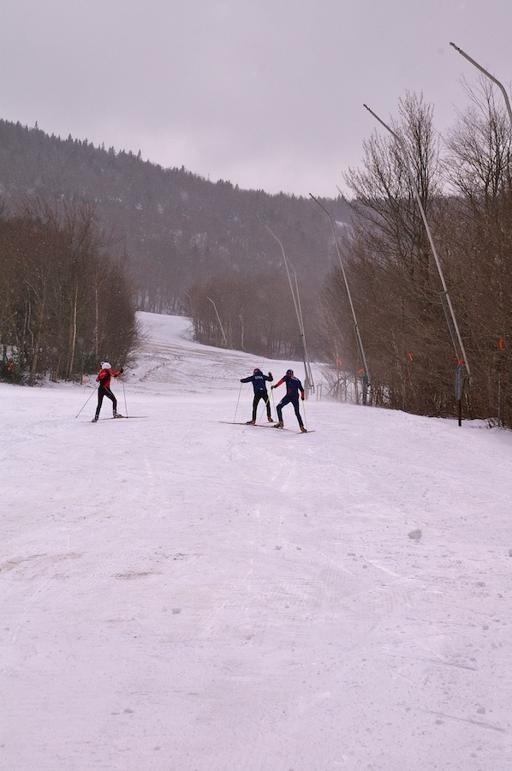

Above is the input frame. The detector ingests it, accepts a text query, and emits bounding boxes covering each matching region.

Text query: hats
[101,363,111,369]
[286,370,294,376]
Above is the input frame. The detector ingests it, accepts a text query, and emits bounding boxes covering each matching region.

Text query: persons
[240,368,274,425]
[272,368,308,434]
[90,361,123,423]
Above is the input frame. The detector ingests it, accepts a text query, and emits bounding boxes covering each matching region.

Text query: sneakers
[300,426,307,432]
[274,423,283,427]
[247,420,255,424]
[268,417,273,422]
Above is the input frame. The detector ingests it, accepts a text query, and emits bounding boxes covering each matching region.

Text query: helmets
[254,368,260,374]
